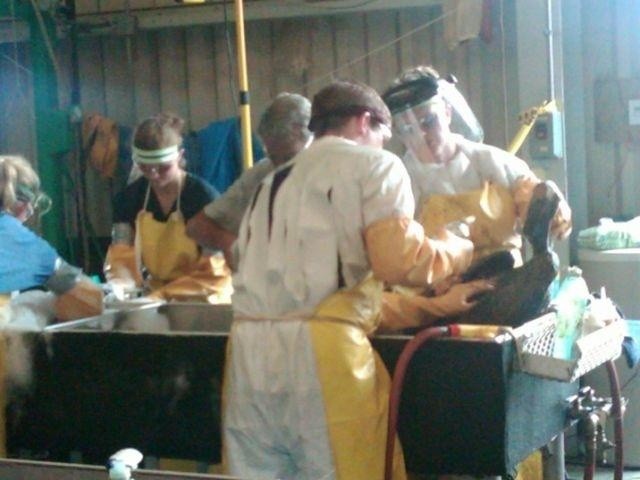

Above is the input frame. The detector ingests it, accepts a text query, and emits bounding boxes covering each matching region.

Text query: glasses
[137,159,173,176]
[20,189,51,218]
[395,112,436,136]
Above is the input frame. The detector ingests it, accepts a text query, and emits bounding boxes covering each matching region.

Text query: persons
[216,73,505,479]
[101,112,232,312]
[368,58,578,301]
[0,152,106,461]
[181,91,318,278]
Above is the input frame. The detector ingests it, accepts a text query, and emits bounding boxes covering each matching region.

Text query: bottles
[549,264,591,360]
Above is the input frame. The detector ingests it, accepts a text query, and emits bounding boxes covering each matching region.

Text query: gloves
[515,179,572,241]
[374,280,494,334]
[363,216,474,289]
